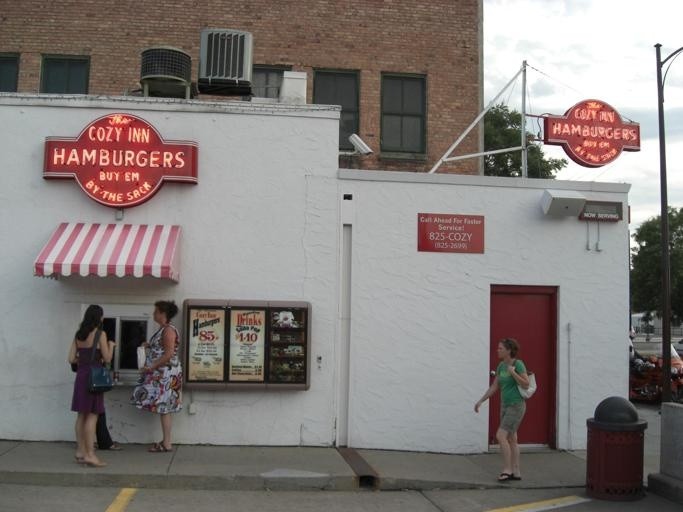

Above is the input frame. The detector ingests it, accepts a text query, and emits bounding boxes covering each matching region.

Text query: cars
[629,337,683,402]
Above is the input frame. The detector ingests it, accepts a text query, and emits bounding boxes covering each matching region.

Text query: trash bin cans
[585,394,648,504]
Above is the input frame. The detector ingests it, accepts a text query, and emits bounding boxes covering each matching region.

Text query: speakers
[539,189,587,218]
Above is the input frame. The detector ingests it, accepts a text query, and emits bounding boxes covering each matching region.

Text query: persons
[68,304,117,467]
[473,339,530,481]
[130,300,185,451]
[94,321,122,450]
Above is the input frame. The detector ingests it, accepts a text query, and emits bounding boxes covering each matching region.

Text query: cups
[113,371,119,383]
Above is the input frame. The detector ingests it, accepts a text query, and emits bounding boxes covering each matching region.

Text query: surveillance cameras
[339,133,374,157]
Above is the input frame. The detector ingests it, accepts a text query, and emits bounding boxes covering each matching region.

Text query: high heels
[75,451,108,468]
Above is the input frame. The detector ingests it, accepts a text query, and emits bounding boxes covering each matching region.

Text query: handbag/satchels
[88,366,113,392]
[517,372,537,399]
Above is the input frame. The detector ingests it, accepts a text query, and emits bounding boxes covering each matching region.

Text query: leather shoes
[109,443,123,450]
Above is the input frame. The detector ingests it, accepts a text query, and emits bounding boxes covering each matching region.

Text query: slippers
[149,440,172,452]
[498,472,521,481]
